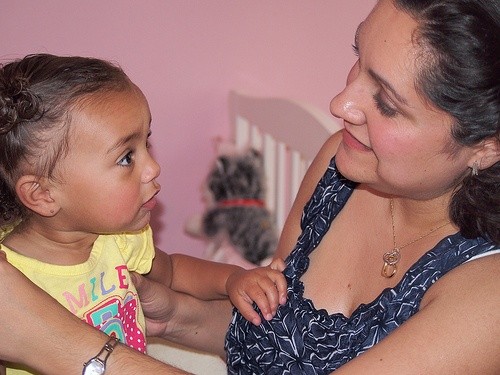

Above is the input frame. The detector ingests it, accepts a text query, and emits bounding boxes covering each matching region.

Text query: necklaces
[379,194,456,279]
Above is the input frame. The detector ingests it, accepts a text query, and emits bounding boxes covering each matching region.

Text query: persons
[0,1,500,375]
[2,53,290,375]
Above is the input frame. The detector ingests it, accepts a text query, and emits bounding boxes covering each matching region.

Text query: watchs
[81,337,122,374]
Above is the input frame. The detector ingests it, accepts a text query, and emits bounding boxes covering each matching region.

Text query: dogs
[185,147,279,267]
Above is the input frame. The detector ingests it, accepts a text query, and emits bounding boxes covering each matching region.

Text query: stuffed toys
[185,140,281,271]
